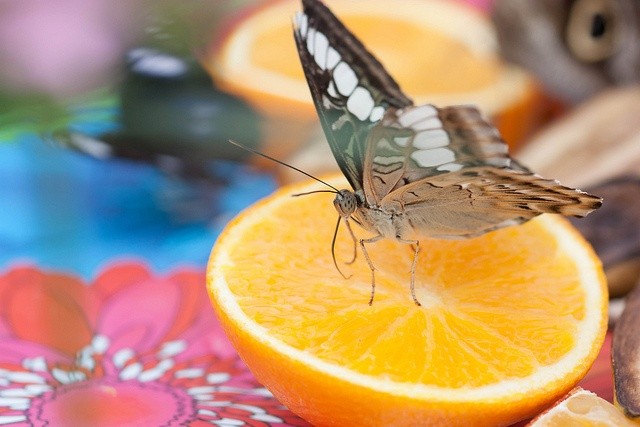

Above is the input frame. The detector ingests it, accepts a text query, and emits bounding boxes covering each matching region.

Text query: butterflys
[227,0,602,309]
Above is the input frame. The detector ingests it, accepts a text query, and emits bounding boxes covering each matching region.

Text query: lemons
[530,382,637,427]
